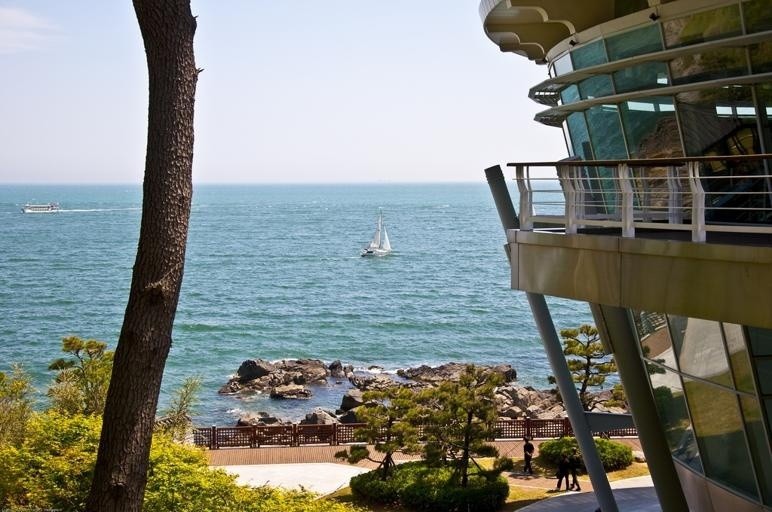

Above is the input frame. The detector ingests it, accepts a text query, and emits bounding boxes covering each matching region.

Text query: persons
[523,437,535,476]
[568,447,582,492]
[555,454,570,492]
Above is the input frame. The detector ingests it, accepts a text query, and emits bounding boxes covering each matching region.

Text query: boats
[20,203,58,213]
[358,207,393,258]
